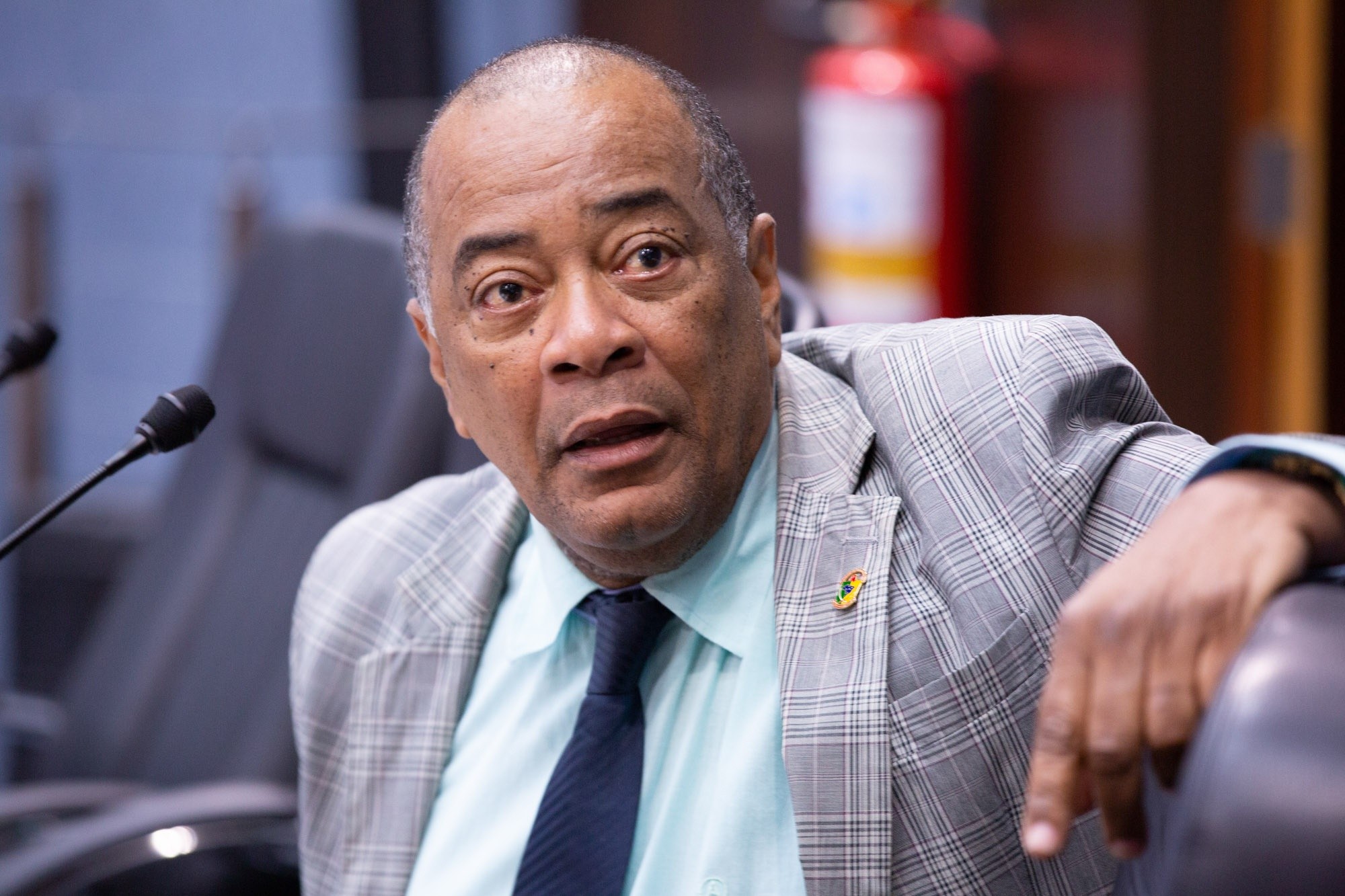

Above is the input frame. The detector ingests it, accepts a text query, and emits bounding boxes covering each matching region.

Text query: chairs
[0,182,490,896]
[1118,574,1345,895]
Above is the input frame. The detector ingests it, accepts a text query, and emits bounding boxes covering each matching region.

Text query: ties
[512,590,677,895]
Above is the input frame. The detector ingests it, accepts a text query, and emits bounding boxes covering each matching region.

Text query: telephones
[0,779,298,896]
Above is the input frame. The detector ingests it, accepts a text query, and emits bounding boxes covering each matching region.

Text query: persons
[285,37,1345,896]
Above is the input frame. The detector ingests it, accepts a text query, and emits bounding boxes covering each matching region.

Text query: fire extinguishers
[798,0,1001,327]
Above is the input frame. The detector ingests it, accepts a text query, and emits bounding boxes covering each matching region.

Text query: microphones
[1,384,217,558]
[0,320,58,384]
